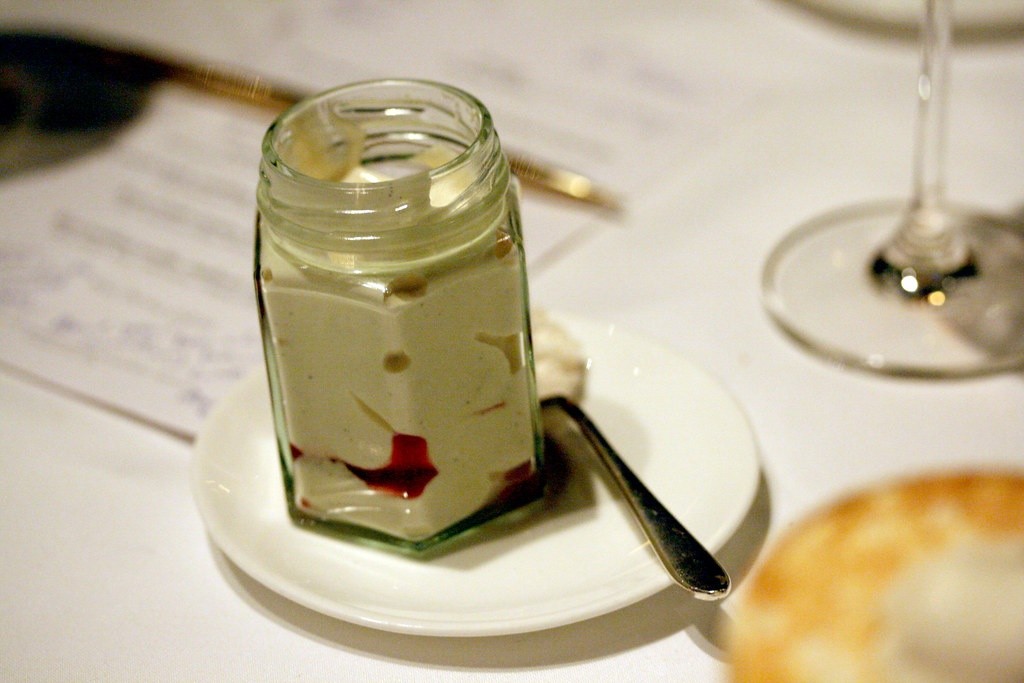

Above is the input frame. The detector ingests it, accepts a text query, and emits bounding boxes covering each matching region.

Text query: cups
[249,77,551,551]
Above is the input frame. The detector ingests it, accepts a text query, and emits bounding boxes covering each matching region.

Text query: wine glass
[760,0,1024,377]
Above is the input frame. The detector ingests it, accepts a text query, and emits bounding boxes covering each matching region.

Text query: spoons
[529,313,729,601]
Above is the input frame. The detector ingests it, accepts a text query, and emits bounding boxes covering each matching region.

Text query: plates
[192,324,762,639]
